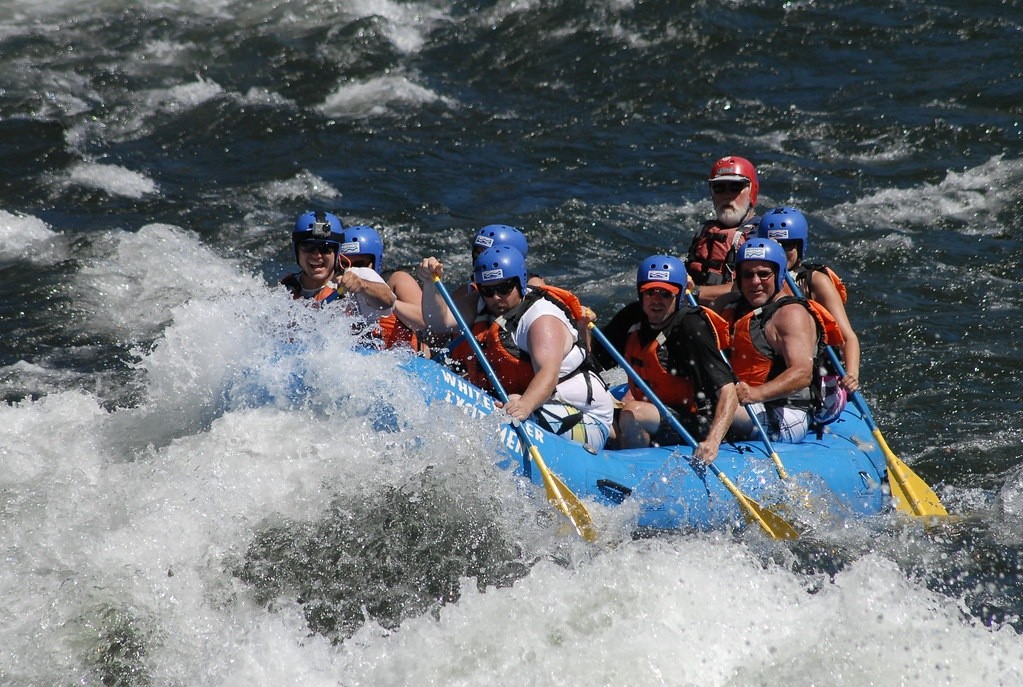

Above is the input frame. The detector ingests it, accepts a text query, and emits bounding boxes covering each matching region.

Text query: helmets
[471,225,528,261]
[637,255,688,311]
[474,244,526,297]
[735,238,787,291]
[291,211,344,258]
[708,156,759,207]
[758,207,808,260]
[335,225,383,275]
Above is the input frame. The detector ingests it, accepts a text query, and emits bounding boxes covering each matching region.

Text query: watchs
[691,286,700,298]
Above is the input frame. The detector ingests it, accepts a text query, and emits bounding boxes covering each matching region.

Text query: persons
[416,244,615,450]
[421,225,547,382]
[730,238,845,443]
[339,226,425,354]
[680,157,759,327]
[758,206,861,393]
[572,255,738,466]
[281,212,397,350]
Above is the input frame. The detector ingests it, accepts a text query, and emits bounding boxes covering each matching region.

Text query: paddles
[429,262,597,542]
[583,308,802,546]
[684,286,811,518]
[783,270,949,517]
[320,286,346,306]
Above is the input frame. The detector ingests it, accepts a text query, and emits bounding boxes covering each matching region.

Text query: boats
[201,335,891,629]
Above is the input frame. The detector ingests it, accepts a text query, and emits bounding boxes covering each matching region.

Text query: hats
[298,234,337,243]
[640,283,680,294]
[710,175,751,182]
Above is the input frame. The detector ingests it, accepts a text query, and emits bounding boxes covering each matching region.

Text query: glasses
[741,270,775,281]
[779,240,798,252]
[479,278,517,297]
[297,242,337,255]
[340,258,374,271]
